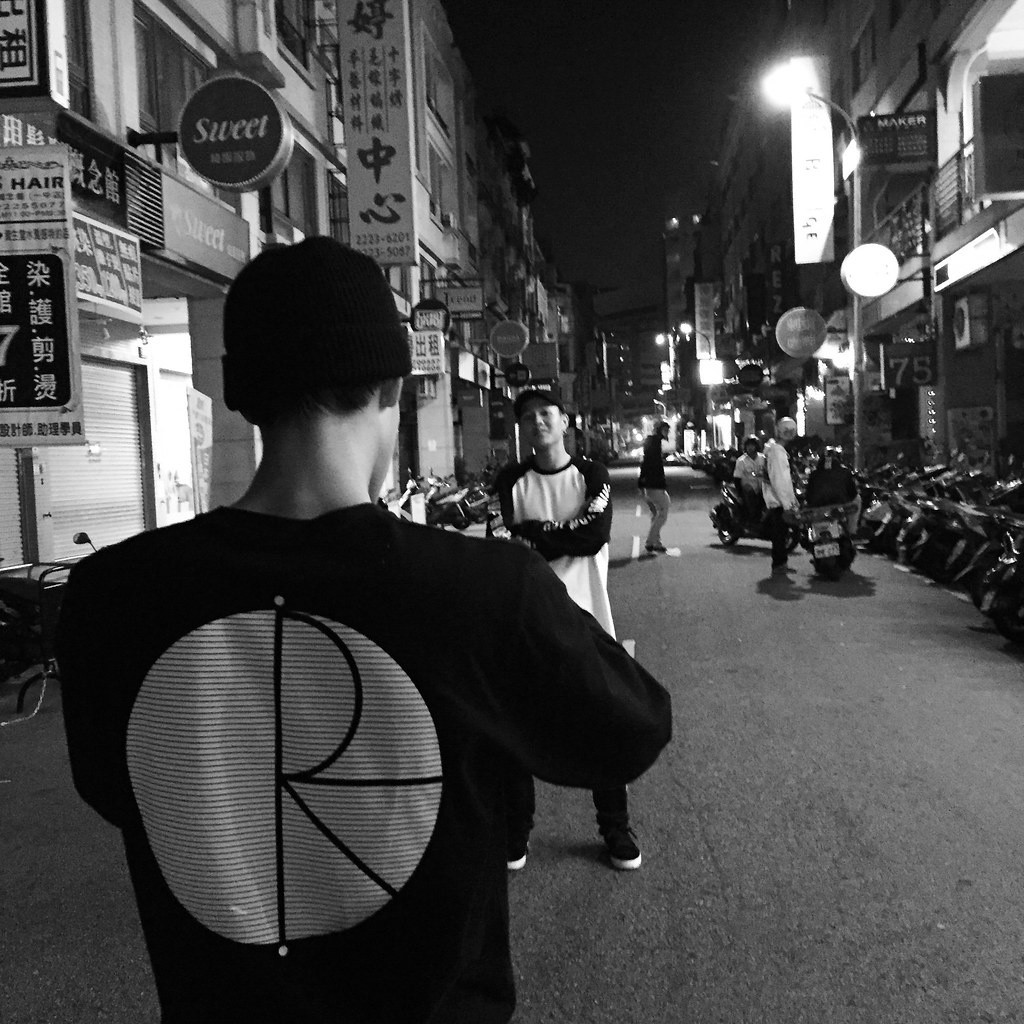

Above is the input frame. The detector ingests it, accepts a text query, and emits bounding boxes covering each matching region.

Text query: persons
[639,421,676,554]
[733,434,763,513]
[807,450,861,535]
[484,381,642,870]
[58,236,672,1024]
[761,417,800,584]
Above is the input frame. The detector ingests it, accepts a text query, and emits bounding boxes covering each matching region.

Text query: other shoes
[644,541,666,553]
[773,565,796,574]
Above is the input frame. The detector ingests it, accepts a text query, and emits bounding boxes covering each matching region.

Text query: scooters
[374,457,502,532]
[699,434,1024,648]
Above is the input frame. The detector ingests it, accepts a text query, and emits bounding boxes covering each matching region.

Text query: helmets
[744,435,761,451]
[776,417,796,440]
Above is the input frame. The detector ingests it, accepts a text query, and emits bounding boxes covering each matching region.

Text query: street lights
[763,61,868,486]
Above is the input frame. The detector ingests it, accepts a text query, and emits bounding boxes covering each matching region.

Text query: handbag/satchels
[804,453,857,507]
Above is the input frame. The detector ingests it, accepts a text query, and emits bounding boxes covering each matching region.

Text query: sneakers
[507,847,528,870]
[605,827,641,870]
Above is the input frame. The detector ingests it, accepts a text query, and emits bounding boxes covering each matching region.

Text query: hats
[513,390,564,415]
